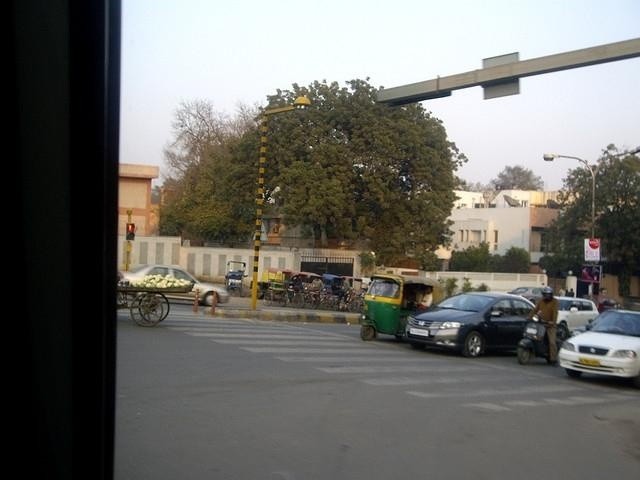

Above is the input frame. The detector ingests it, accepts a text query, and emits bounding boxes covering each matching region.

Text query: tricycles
[118,279,196,330]
[260,268,368,313]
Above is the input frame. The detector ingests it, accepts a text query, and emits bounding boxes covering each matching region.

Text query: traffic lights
[125,222,136,242]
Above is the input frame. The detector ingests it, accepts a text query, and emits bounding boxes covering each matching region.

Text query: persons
[310,277,325,292]
[525,286,559,364]
[416,286,433,310]
[341,276,351,288]
[558,286,575,297]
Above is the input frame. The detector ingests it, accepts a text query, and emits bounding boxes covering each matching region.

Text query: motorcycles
[513,312,566,371]
[356,271,443,342]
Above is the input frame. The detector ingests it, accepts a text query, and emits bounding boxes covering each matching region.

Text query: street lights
[540,152,598,275]
[250,93,315,310]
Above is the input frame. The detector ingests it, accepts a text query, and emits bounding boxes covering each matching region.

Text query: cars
[117,262,232,309]
[555,307,640,387]
[400,289,537,360]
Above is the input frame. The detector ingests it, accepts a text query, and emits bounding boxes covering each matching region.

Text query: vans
[504,285,542,297]
[532,296,601,332]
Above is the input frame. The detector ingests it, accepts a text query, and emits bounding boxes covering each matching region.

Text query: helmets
[542,286,554,295]
